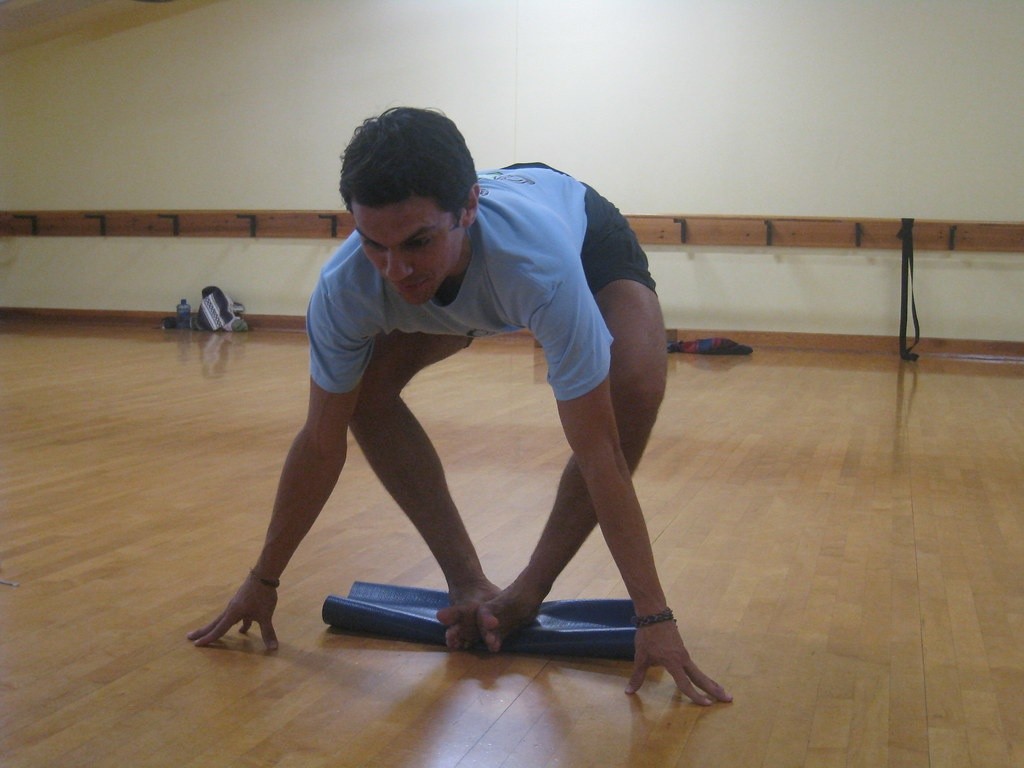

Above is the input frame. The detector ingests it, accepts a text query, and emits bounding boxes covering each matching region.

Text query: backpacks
[195,286,244,332]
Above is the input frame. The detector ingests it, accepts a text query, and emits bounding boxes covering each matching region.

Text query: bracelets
[631,608,676,628]
[250,566,282,588]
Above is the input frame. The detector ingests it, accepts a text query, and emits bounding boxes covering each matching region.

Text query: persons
[187,107,735,709]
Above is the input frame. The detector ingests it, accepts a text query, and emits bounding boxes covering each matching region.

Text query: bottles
[177,300,190,329]
